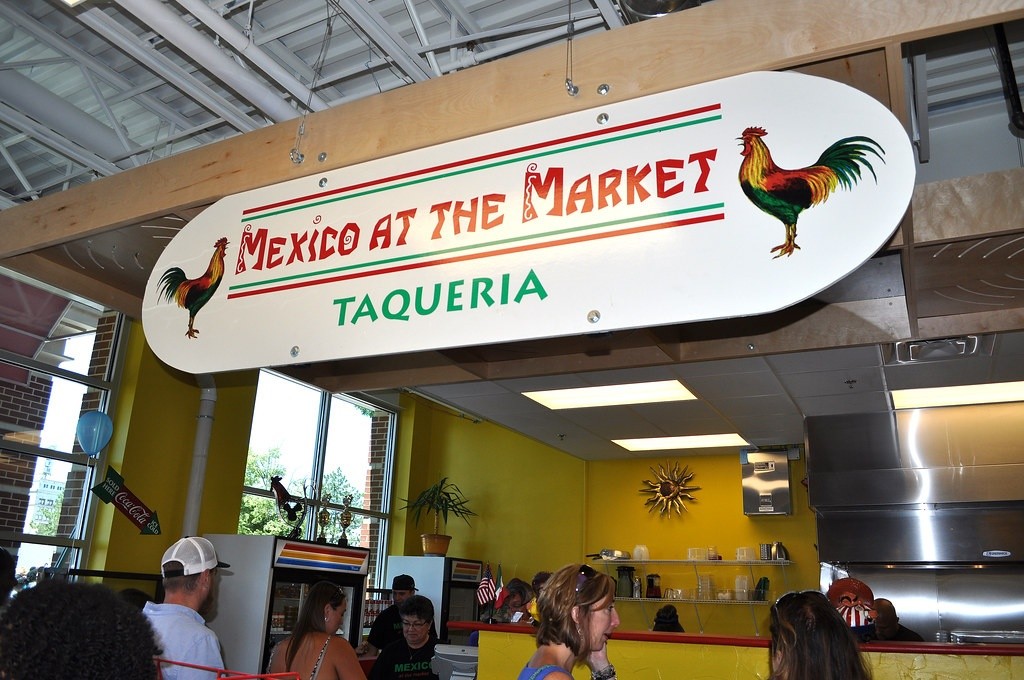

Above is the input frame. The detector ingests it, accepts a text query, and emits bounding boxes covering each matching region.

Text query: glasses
[575,565,594,592]
[330,587,343,602]
[402,621,427,628]
[775,589,830,627]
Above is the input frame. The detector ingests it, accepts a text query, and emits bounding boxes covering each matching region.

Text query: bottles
[776,542,790,564]
[632,544,649,559]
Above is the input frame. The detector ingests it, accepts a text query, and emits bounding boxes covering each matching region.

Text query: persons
[654,605,685,632]
[357,574,442,680]
[9,566,51,600]
[265,581,367,680]
[766,589,873,680]
[468,571,553,646]
[0,579,162,680]
[141,536,230,680]
[0,547,18,604]
[517,564,620,680]
[855,598,924,642]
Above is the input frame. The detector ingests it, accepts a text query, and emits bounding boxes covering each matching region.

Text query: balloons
[77,411,113,456]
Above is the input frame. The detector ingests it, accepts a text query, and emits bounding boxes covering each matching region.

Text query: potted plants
[399,478,478,557]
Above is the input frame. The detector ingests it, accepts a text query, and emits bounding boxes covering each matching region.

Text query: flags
[478,566,510,609]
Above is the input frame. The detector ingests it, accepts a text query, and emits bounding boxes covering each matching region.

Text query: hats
[392,574,419,591]
[161,535,230,577]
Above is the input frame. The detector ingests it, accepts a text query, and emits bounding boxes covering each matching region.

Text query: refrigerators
[197,533,372,680]
[384,555,484,647]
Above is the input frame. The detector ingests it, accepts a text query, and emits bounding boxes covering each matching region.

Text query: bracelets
[591,664,617,680]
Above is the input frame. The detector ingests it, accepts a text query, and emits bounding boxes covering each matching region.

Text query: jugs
[647,573,662,598]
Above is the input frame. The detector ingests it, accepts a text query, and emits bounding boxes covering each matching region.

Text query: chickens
[735,126,886,259]
[156,236,230,338]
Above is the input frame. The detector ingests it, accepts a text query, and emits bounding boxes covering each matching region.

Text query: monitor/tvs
[431,644,478,680]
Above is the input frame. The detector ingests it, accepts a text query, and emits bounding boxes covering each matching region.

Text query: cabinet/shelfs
[589,558,789,605]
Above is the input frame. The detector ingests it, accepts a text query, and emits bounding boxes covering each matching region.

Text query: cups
[689,548,702,560]
[734,574,749,601]
[707,546,718,560]
[759,543,774,560]
[615,566,636,598]
[697,574,717,600]
